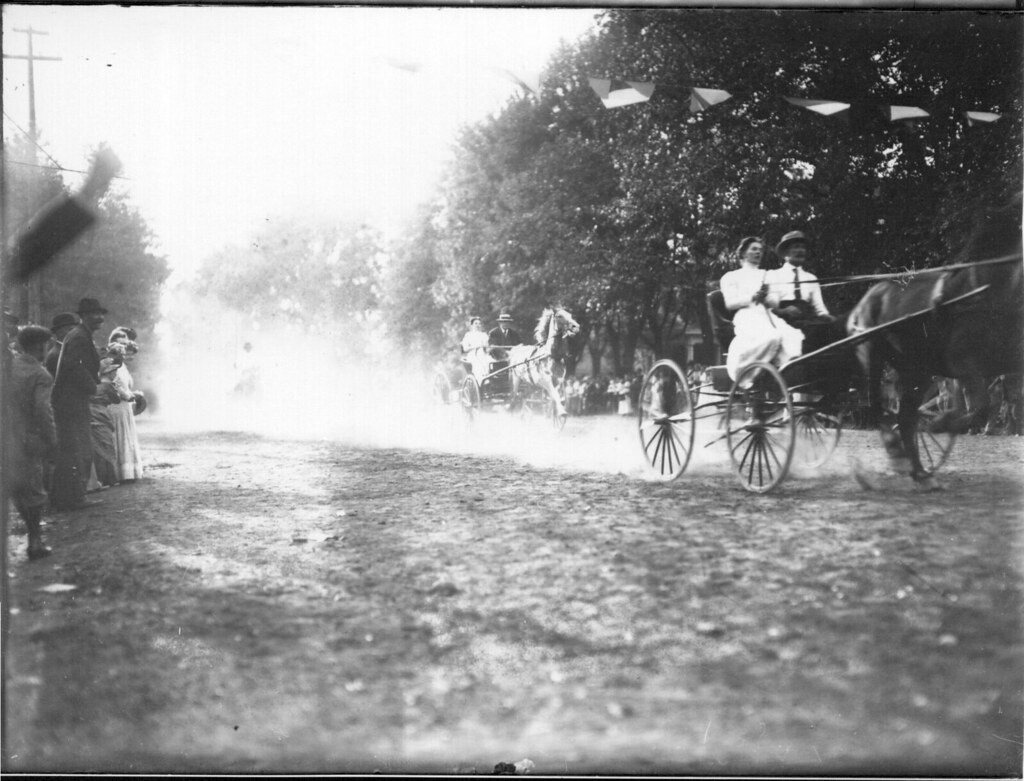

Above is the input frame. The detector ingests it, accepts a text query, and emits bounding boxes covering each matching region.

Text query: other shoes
[72,499,104,510]
[88,485,111,494]
[120,478,136,484]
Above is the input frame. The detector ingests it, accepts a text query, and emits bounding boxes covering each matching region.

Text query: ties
[792,268,801,300]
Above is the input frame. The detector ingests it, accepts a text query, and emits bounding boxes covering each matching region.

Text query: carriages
[637,245,1023,492]
[459,303,589,431]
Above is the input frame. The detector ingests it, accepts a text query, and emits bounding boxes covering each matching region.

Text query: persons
[526,360,719,417]
[459,314,524,387]
[0,296,144,559]
[719,230,846,390]
[745,361,1024,437]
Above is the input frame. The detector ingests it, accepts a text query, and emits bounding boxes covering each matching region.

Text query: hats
[50,313,79,333]
[99,357,120,374]
[18,324,52,346]
[776,230,808,260]
[496,313,513,322]
[131,391,146,415]
[75,297,108,314]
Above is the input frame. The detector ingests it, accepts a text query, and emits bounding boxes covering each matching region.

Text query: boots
[21,506,51,561]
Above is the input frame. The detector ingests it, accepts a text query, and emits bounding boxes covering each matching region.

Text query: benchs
[706,290,736,363]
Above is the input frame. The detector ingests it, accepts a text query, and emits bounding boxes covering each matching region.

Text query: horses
[508,304,580,418]
[845,190,1024,487]
